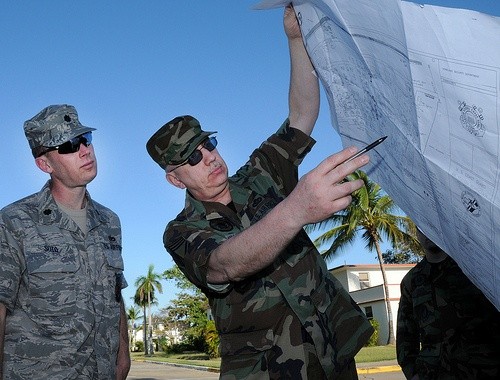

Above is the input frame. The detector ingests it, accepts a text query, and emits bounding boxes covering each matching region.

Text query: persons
[396,224,500,380]
[0,104,132,380]
[145,1,375,380]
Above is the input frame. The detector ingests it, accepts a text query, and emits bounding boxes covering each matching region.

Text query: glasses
[168,136,218,173]
[36,132,94,156]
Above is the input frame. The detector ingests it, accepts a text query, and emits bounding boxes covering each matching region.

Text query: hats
[23,103,98,157]
[145,116,219,169]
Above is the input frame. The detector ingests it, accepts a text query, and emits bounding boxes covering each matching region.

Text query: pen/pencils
[335,135,388,168]
